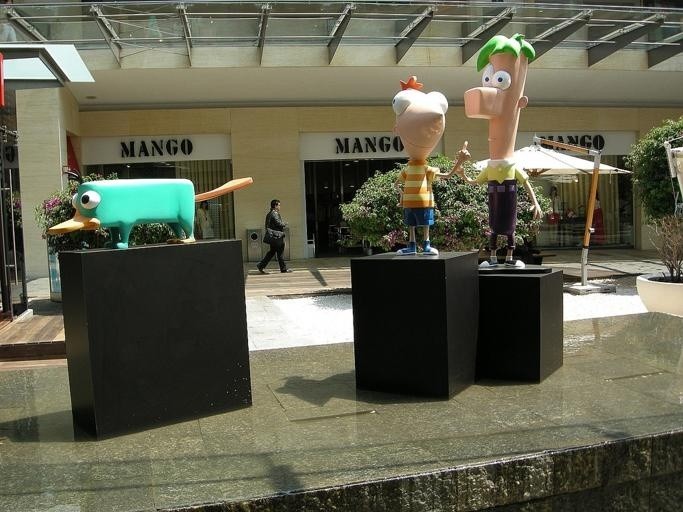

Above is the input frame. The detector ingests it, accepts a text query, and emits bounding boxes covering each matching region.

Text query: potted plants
[625,114,683,317]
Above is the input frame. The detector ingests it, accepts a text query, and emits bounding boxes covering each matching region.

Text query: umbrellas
[473,141,634,185]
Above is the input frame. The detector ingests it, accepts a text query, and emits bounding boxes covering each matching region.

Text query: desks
[558,216,594,247]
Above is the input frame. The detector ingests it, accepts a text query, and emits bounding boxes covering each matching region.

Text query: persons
[256,199,292,275]
[588,190,605,245]
[547,186,563,247]
[196,200,215,239]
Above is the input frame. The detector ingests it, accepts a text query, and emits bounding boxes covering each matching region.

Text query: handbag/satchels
[263,228,285,248]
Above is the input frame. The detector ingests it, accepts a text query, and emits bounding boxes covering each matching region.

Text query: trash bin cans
[275,227,290,261]
[247,228,263,262]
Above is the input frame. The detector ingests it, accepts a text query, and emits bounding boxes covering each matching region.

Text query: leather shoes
[257,263,265,273]
[281,269,291,272]
